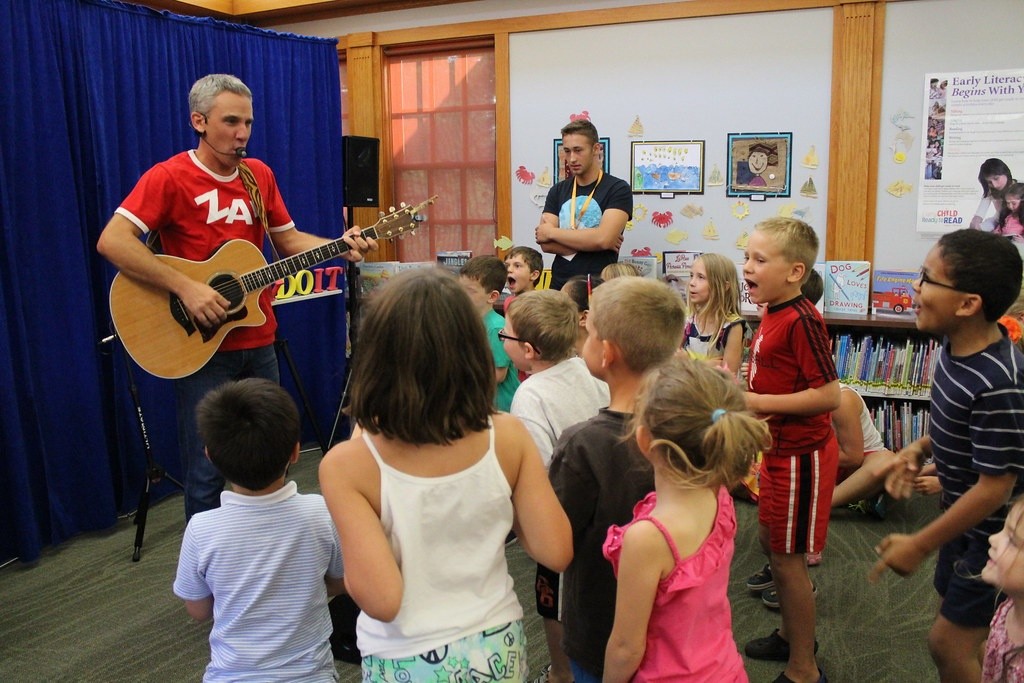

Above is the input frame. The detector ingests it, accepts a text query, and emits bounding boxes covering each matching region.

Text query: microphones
[196,129,247,158]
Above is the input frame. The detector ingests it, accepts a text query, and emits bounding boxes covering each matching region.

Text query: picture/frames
[630,140,705,195]
[726,132,792,201]
[553,136,610,185]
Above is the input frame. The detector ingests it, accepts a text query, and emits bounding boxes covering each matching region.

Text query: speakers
[341,135,380,208]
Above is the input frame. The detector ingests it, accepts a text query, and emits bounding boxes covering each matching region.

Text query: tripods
[121,347,187,562]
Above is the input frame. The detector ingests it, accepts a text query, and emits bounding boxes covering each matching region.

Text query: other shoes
[807,550,821,566]
[503,530,518,549]
[528,663,551,683]
[849,492,887,519]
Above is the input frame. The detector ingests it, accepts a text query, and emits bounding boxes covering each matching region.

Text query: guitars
[108,201,420,381]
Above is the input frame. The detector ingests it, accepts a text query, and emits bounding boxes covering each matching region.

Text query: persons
[535,119,633,291]
[969,158,1024,261]
[498,289,611,468]
[827,383,899,522]
[97,74,380,525]
[173,377,344,683]
[602,351,772,683]
[869,229,1024,682]
[457,247,824,413]
[319,265,574,683]
[743,216,843,683]
[981,496,1024,683]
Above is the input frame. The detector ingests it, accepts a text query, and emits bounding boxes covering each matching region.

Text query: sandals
[745,628,817,661]
[772,668,827,682]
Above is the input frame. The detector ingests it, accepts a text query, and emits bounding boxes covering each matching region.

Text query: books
[824,334,943,467]
[872,267,921,320]
[825,260,871,316]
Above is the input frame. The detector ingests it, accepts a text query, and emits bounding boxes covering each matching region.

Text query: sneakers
[749,565,774,590]
[761,579,817,608]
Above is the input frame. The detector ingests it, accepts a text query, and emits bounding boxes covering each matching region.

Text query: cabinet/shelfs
[737,312,943,465]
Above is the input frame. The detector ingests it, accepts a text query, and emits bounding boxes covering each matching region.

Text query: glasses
[917,264,977,294]
[497,329,541,355]
